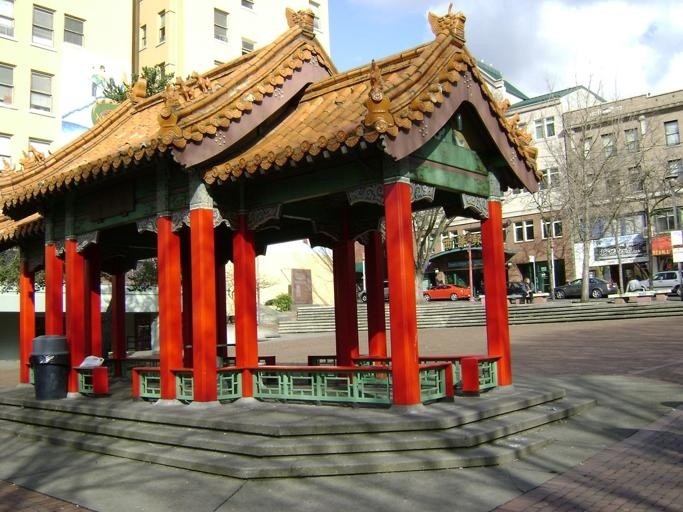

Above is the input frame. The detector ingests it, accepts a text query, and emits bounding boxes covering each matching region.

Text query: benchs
[478,292,551,305]
[607,288,672,304]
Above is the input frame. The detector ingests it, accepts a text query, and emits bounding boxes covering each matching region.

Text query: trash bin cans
[29,335,69,400]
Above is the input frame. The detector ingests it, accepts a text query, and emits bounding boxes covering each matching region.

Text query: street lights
[663,171,682,297]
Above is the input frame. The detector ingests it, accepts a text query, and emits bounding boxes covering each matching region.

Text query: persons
[625,276,645,293]
[521,277,534,304]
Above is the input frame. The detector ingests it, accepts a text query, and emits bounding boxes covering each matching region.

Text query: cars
[358,280,389,304]
[553,277,617,299]
[422,283,470,302]
[638,269,682,299]
[506,281,533,297]
